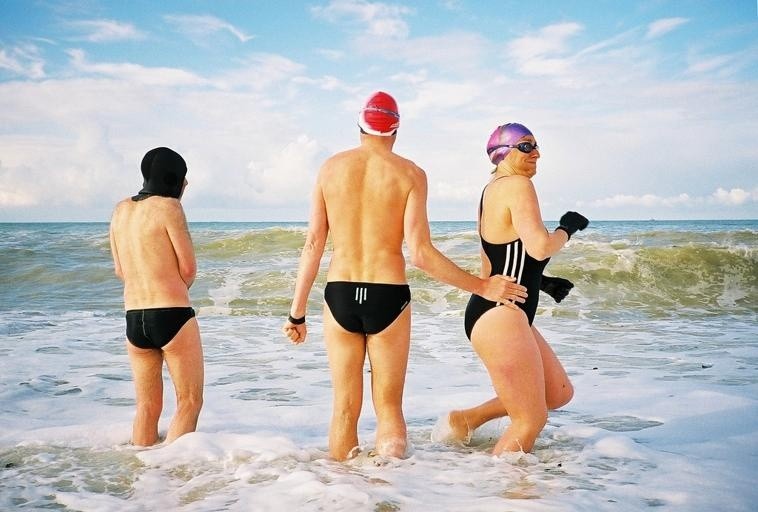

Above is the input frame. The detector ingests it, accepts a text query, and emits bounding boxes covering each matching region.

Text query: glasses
[486,142,538,154]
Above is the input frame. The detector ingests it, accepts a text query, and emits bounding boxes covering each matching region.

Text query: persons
[109,147,204,447]
[448,123,589,456]
[283,91,528,461]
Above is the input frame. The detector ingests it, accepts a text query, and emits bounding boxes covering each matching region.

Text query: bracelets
[287,315,305,324]
